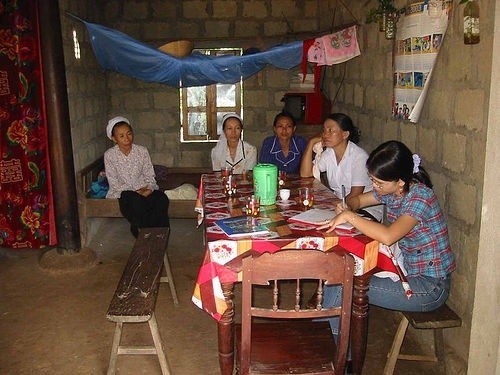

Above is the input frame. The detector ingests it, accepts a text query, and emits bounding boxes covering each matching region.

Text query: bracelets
[351,215,360,225]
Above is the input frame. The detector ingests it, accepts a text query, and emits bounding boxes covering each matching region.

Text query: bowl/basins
[158,40,194,57]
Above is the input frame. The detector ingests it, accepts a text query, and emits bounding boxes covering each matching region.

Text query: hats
[107,116,130,140]
[222,113,243,130]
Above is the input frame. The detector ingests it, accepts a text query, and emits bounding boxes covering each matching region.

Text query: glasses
[367,171,388,189]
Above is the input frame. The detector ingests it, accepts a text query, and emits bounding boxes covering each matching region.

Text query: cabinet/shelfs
[292,123,323,140]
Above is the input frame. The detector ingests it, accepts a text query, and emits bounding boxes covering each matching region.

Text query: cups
[223,176,236,196]
[246,194,260,216]
[279,189,290,200]
[299,187,314,210]
[221,167,233,180]
[278,170,286,189]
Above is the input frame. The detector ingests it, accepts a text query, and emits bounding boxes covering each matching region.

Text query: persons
[104,116,170,238]
[300,113,384,223]
[211,113,257,174]
[259,112,308,174]
[315,140,456,375]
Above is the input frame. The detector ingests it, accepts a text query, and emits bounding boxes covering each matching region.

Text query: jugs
[252,163,278,205]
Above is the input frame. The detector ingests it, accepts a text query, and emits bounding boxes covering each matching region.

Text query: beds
[76,157,213,249]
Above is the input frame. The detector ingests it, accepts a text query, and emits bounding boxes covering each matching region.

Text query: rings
[327,223,331,227]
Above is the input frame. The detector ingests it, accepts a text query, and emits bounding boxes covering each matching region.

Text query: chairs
[235,250,355,375]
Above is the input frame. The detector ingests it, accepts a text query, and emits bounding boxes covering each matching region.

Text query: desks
[193,172,379,375]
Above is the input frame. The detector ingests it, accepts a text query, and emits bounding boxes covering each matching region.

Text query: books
[287,73,315,93]
[214,215,269,237]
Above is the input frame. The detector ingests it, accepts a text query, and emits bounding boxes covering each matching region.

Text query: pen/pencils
[342,184,346,209]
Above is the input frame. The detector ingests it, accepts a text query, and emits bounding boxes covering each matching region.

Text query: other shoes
[306,289,319,309]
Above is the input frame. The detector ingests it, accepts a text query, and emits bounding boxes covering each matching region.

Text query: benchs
[104,227,179,375]
[382,305,462,375]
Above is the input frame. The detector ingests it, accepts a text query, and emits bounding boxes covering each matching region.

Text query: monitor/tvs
[283,91,330,125]
